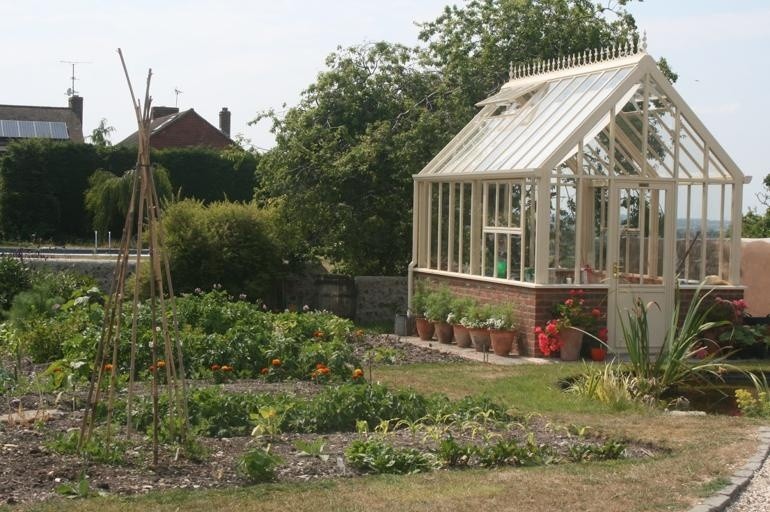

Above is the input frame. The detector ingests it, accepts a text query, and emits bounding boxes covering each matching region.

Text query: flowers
[530,289,606,357]
[442,300,511,333]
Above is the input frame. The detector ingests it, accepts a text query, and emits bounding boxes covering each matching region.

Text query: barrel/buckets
[316,273,356,318]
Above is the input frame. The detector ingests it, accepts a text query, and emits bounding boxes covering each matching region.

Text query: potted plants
[404,283,451,343]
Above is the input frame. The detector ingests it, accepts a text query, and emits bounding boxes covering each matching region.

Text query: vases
[452,323,516,355]
[558,326,584,361]
[588,347,605,361]
